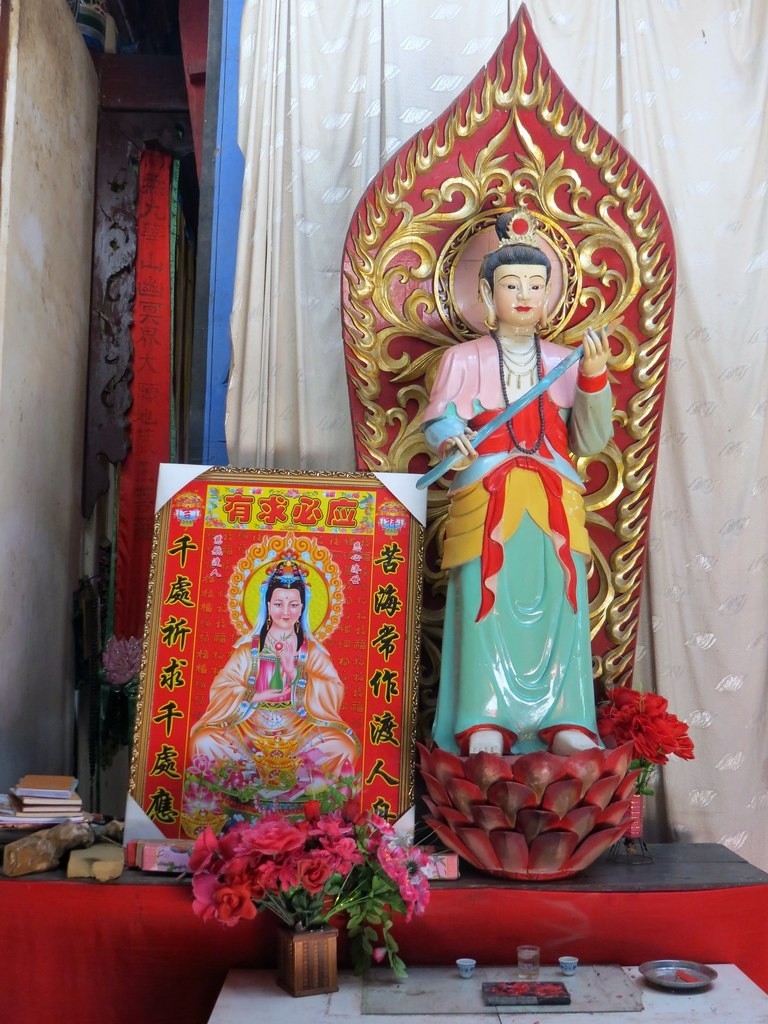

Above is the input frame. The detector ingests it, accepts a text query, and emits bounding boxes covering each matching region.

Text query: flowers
[598,686,695,796]
[189,798,430,977]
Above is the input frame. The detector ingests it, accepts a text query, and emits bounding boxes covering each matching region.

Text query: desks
[208,964,768,1024]
[0,843,768,1024]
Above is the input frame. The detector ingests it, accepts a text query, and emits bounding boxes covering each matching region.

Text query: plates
[638,959,719,989]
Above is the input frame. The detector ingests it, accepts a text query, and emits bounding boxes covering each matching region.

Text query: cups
[558,956,579,975]
[516,945,540,980]
[456,958,476,979]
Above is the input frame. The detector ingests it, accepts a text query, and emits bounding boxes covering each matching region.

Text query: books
[0,774,86,824]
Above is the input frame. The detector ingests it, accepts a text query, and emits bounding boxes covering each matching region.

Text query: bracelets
[251,703,260,709]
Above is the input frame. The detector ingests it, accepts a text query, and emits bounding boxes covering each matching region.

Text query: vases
[274,921,338,998]
[606,795,655,865]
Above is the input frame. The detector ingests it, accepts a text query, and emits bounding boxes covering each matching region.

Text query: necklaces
[489,328,544,454]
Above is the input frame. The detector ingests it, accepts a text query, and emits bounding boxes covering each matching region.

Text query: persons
[193,559,357,800]
[421,209,612,757]
